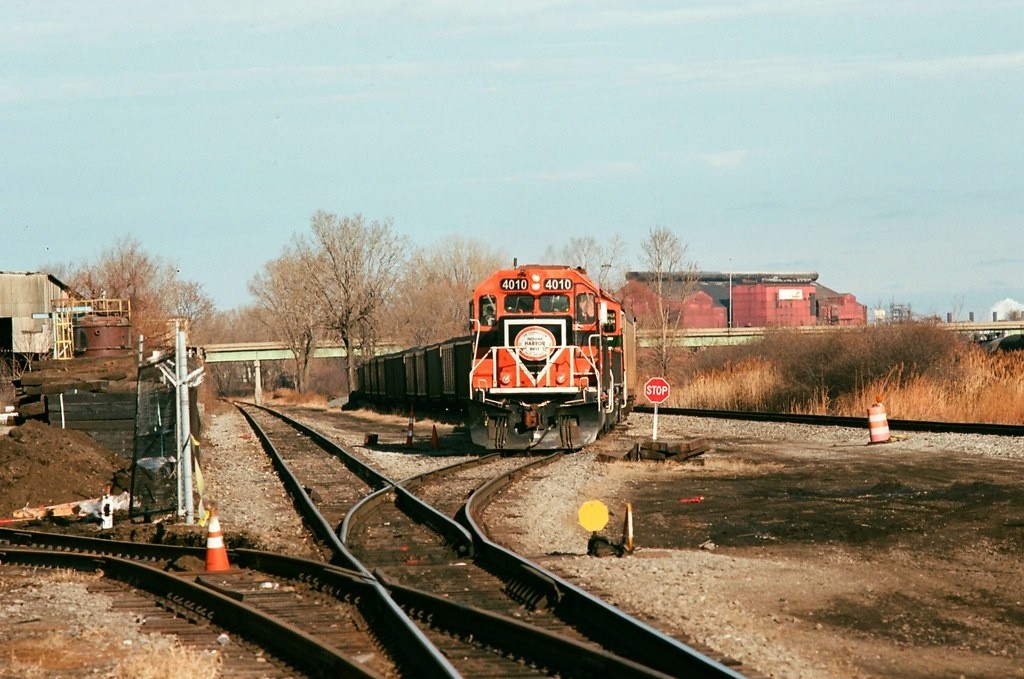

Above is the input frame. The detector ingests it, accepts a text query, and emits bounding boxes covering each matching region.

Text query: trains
[356,258,629,459]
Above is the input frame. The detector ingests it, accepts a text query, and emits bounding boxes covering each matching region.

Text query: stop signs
[644,377,671,403]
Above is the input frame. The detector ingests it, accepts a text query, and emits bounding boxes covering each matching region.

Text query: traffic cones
[204,507,231,572]
[430,424,441,449]
